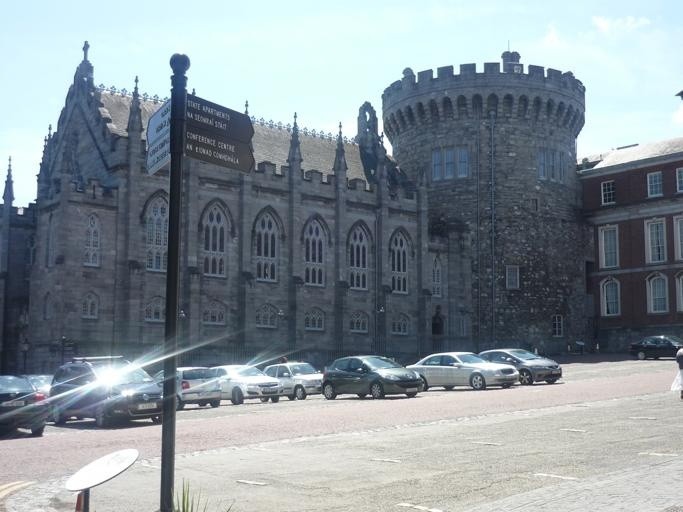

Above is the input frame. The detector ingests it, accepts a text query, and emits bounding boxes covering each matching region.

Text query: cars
[211,363,285,406]
[321,355,424,399]
[404,351,520,391]
[21,373,55,421]
[260,361,324,403]
[1,374,51,438]
[154,366,222,411]
[478,347,562,386]
[51,355,164,429]
[628,334,683,360]
